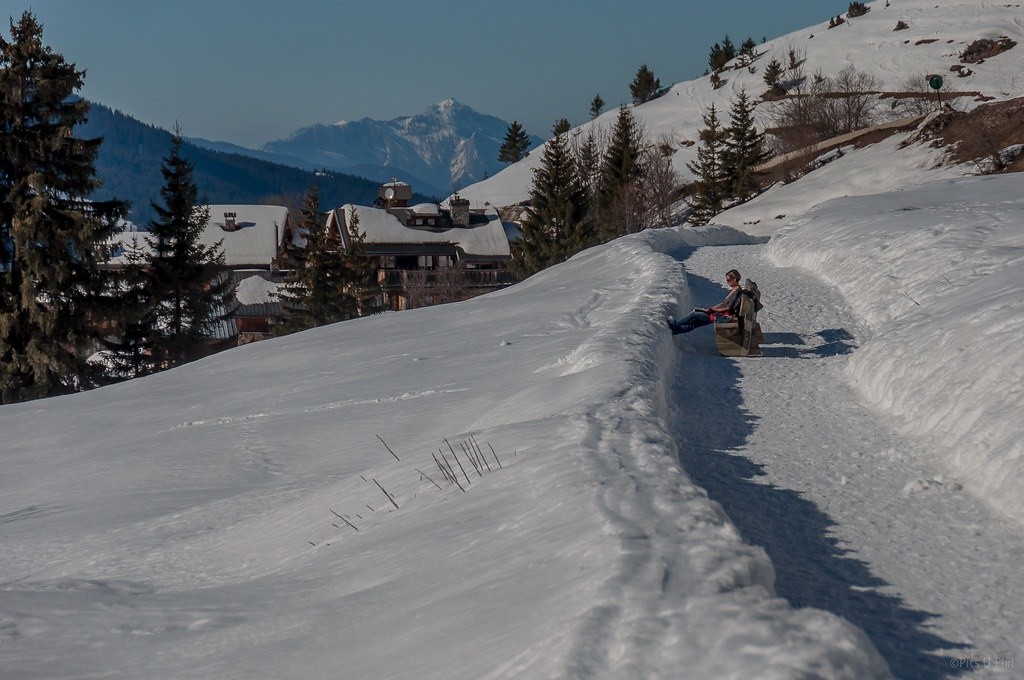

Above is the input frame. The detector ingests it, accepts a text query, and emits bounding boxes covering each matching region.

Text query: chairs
[714,279,765,357]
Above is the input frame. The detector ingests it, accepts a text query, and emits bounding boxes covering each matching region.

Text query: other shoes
[667,315,676,334]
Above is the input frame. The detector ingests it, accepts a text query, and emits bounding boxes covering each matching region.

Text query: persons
[668,269,741,335]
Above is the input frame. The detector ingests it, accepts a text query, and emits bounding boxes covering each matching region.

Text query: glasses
[727,277,731,282]
